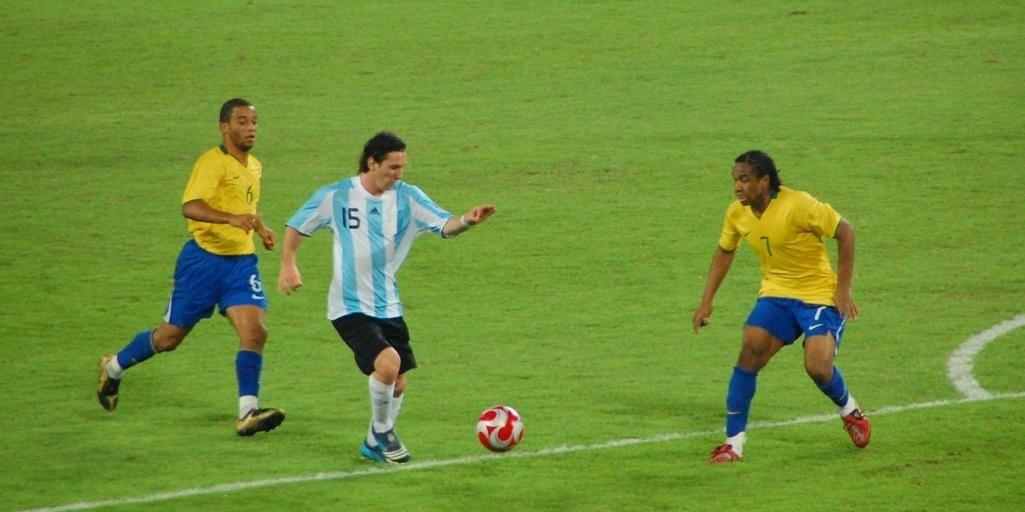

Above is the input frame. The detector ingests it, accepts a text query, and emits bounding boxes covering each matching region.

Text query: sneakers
[235,408,285,436]
[840,399,871,448]
[97,354,122,410]
[359,419,410,464]
[705,444,744,463]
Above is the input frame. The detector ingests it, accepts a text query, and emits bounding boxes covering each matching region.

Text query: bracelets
[460,215,470,230]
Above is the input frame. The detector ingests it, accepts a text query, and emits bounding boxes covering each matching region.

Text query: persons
[693,151,871,464]
[279,133,496,465]
[98,99,286,436]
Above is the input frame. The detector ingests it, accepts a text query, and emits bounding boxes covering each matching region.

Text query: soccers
[474,402,524,454]
